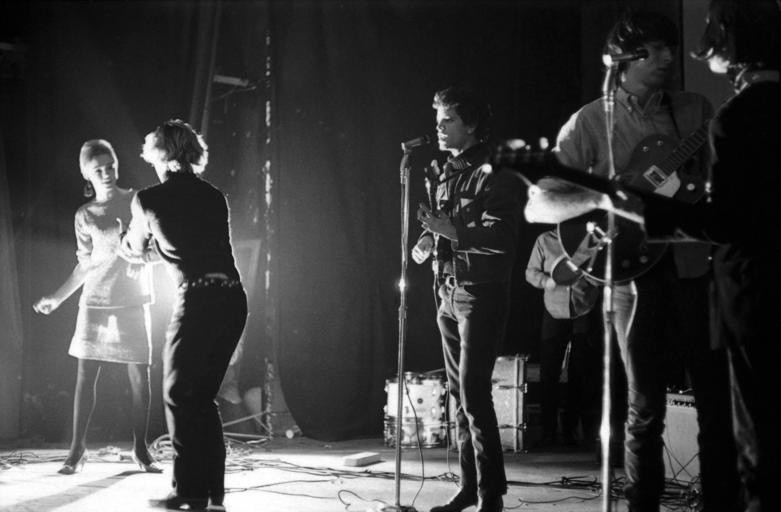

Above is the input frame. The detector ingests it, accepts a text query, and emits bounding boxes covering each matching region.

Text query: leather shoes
[207,486,224,506]
[430,482,478,512]
[480,489,503,511]
[150,489,209,510]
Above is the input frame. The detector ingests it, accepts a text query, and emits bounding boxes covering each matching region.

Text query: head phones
[696,1,731,53]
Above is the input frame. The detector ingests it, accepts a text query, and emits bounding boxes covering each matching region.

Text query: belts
[180,278,244,290]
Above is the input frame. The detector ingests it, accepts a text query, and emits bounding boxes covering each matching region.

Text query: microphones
[401,132,437,149]
[687,51,714,62]
[603,49,650,63]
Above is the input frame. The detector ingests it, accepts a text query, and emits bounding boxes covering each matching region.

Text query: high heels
[57,446,90,478]
[130,448,164,474]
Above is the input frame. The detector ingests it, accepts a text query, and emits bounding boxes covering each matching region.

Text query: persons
[525,229,599,453]
[33,140,164,475]
[609,1,780,510]
[524,17,744,510]
[411,86,525,511]
[117,120,248,510]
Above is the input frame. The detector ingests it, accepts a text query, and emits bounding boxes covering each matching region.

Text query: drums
[383,371,447,449]
[490,350,529,454]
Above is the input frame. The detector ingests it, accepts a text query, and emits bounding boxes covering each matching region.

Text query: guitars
[414,162,445,310]
[556,119,715,282]
[492,136,711,243]
